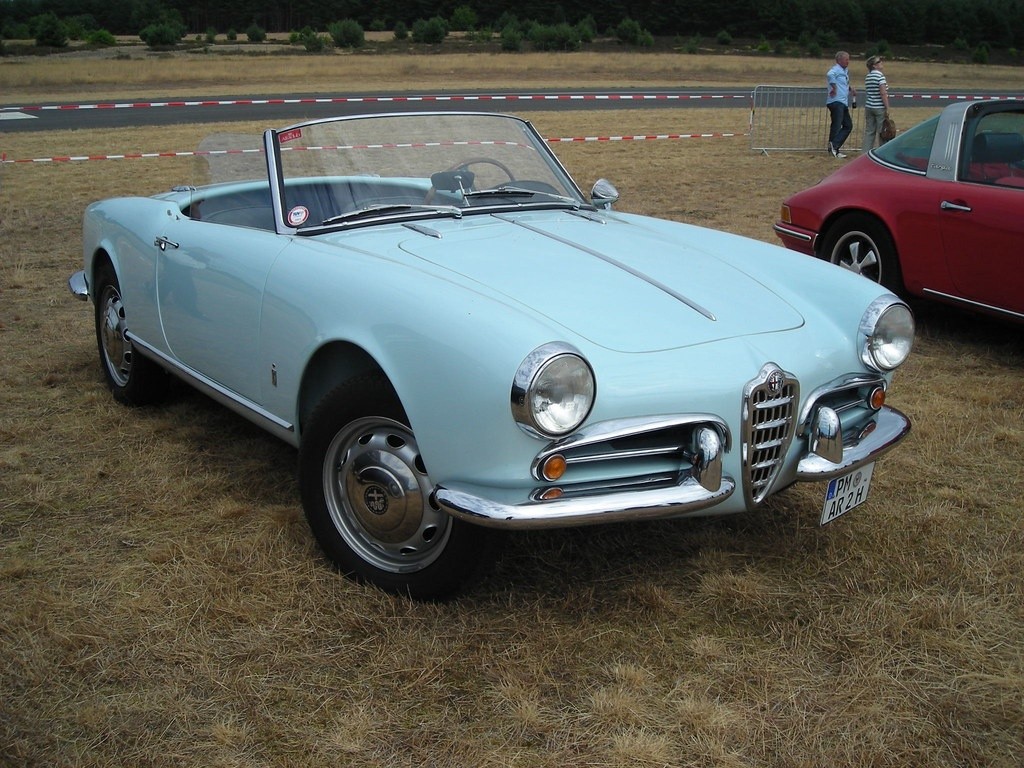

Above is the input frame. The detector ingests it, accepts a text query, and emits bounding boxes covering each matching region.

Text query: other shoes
[837,153,847,158]
[828,142,836,157]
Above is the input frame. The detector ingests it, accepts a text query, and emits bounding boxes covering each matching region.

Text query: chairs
[971,132,1024,188]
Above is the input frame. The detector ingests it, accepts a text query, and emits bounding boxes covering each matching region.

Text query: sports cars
[770,96,1024,318]
[68,112,919,603]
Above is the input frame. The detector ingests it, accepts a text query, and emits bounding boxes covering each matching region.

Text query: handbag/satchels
[881,114,896,141]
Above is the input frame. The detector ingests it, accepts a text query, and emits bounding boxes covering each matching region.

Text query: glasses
[873,60,881,65]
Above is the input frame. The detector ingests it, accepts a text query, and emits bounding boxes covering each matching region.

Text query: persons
[826,51,857,158]
[862,55,889,155]
